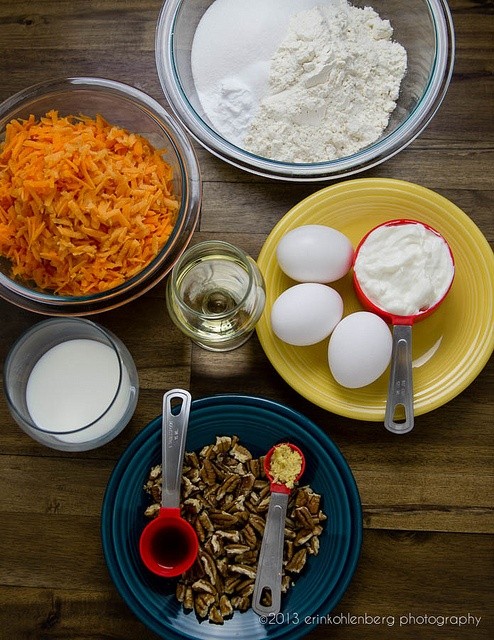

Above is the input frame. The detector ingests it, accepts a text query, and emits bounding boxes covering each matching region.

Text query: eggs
[328,311,394,389]
[276,225,354,284]
[271,283,343,346]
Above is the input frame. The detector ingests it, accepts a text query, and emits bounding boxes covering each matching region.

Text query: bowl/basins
[154,0,456,185]
[0,74,204,318]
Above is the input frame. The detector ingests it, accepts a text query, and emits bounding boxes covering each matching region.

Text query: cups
[2,317,141,453]
[165,237,269,355]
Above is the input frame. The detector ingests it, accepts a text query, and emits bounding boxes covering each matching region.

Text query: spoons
[351,217,457,436]
[138,384,199,581]
[251,438,307,620]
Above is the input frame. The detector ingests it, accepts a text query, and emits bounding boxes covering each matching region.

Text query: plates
[99,392,365,639]
[254,175,494,424]
